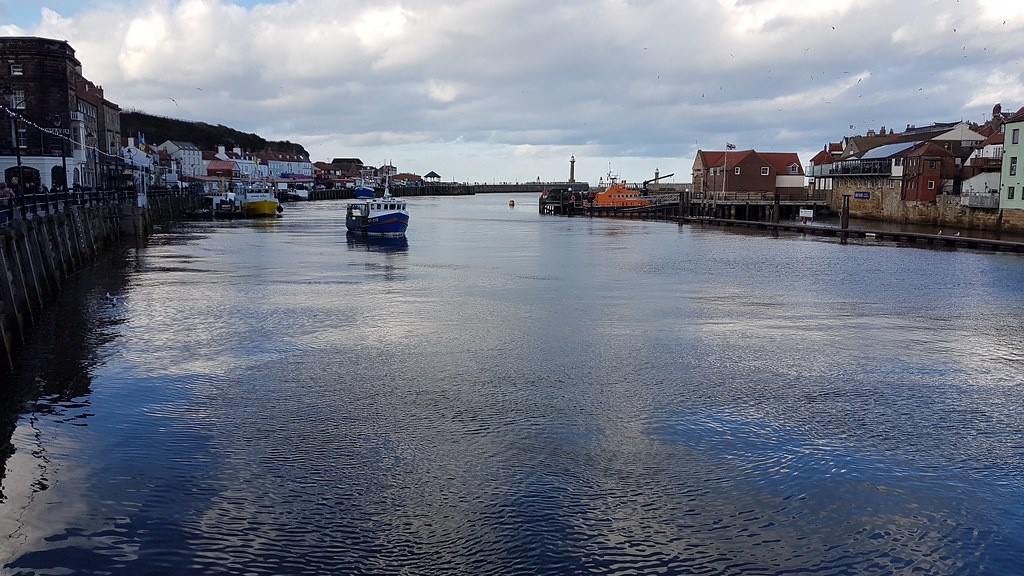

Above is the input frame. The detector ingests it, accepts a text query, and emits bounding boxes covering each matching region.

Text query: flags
[726,142,737,150]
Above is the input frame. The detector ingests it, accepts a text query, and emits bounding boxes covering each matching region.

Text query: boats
[242,199,280,216]
[346,196,409,238]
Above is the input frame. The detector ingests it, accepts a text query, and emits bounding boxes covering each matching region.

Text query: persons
[22,179,116,209]
[9,176,24,220]
[708,190,714,201]
[0,181,15,224]
